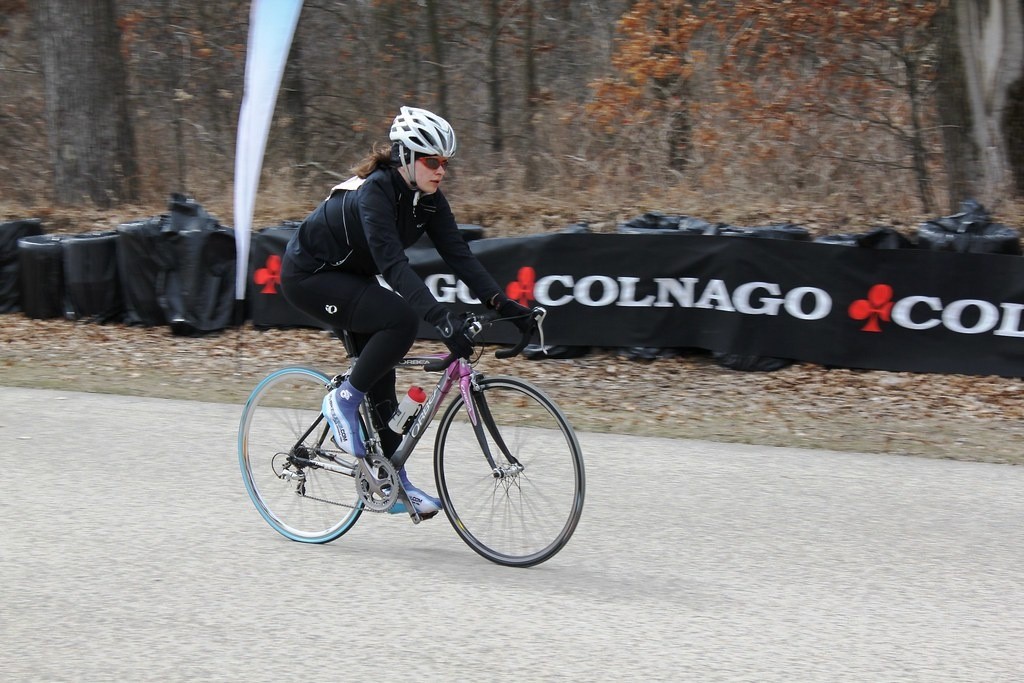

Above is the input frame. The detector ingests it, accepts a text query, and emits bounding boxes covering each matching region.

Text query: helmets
[389,106,457,157]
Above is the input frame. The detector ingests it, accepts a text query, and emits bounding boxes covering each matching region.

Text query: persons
[279,106,534,514]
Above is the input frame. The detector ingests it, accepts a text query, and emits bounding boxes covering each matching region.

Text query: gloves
[424,304,474,360]
[490,290,533,334]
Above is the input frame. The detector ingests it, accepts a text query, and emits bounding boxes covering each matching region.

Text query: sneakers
[383,464,445,514]
[322,379,367,460]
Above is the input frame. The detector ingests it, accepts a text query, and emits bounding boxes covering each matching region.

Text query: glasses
[419,154,450,171]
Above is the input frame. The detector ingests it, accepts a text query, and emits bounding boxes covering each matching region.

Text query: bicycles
[234,306,587,568]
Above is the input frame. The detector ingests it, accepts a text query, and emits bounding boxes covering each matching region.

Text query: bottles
[387,386,426,434]
